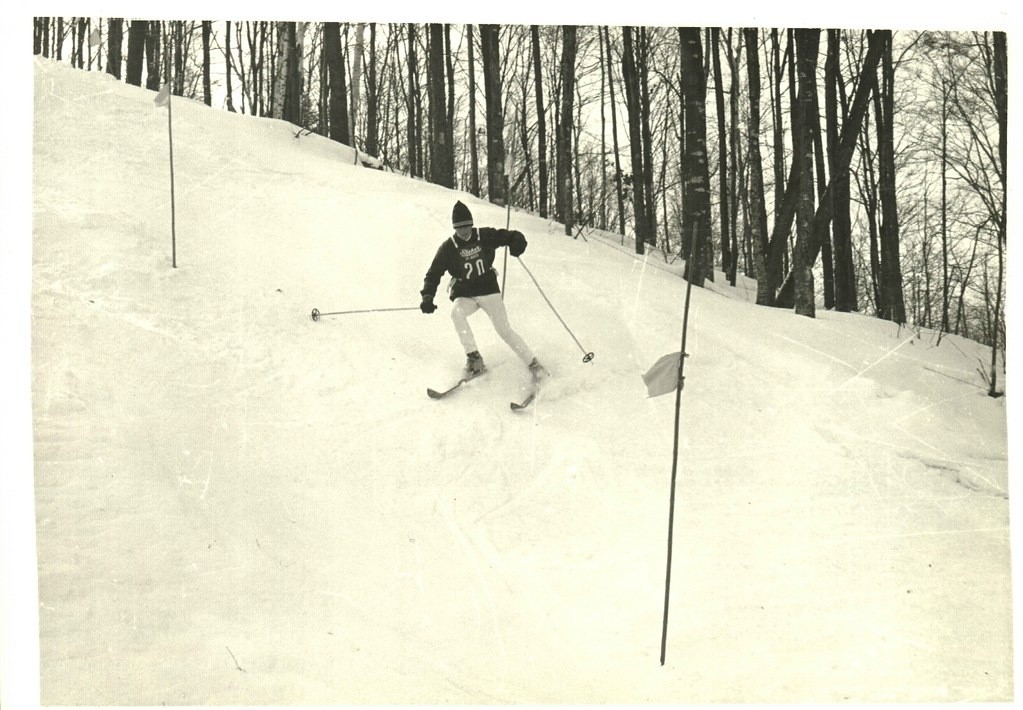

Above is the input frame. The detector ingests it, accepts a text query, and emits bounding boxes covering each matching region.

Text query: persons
[420,200,549,385]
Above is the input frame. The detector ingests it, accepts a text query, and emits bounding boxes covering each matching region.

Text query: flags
[153,82,170,109]
[90,29,100,45]
[641,350,689,397]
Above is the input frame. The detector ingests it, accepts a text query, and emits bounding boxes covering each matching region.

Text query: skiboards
[427,360,548,410]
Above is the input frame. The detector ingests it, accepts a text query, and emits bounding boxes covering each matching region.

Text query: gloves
[510,240,524,257]
[420,295,437,314]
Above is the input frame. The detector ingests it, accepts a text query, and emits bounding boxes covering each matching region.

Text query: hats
[452,200,473,228]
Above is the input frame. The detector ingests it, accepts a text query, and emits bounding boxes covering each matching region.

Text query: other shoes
[528,357,547,387]
[463,350,484,375]
